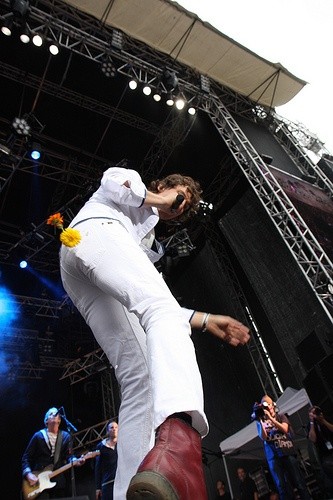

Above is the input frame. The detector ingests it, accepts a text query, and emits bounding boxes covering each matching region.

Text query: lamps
[24,139,46,161]
[1,25,200,116]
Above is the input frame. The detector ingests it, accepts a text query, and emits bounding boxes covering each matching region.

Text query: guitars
[22,449,101,500]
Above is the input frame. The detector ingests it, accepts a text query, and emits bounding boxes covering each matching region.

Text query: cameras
[315,408,320,416]
[253,401,268,419]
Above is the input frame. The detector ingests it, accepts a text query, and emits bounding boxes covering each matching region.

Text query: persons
[256,396,312,500]
[308,406,333,483]
[94,420,118,500]
[216,479,231,500]
[236,466,258,500]
[22,406,85,500]
[59,167,251,500]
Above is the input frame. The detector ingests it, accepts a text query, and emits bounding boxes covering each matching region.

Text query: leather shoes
[127,418,209,500]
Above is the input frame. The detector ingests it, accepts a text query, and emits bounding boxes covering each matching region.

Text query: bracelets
[200,312,210,332]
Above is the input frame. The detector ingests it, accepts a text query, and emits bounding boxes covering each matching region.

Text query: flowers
[47,213,82,248]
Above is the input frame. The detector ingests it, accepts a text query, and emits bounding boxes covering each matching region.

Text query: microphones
[53,407,63,417]
[170,195,184,213]
